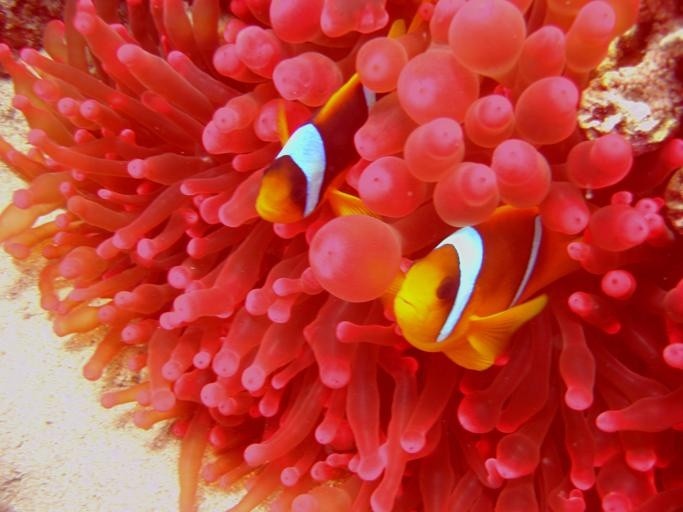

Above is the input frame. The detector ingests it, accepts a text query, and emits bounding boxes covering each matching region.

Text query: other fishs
[255,10,433,224]
[382,204,586,372]
